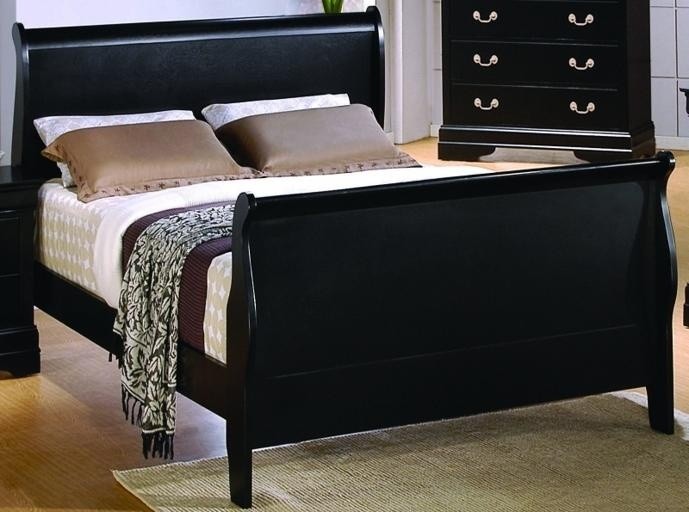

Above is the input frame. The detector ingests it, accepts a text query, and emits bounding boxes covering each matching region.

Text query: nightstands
[0,180,44,380]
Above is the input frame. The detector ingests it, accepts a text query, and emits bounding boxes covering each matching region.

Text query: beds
[9,4,683,509]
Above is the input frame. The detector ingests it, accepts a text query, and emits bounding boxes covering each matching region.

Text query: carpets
[111,384,688,512]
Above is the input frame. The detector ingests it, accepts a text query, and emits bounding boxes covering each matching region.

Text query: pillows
[218,107,415,179]
[198,95,355,128]
[40,122,248,201]
[34,110,191,190]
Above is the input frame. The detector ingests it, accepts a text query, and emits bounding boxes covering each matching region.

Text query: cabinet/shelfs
[435,2,660,166]
